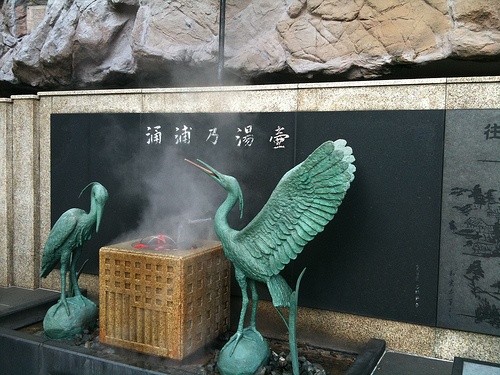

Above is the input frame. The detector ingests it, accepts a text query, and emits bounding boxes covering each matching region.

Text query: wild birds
[183,137,358,358]
[39,182,110,318]
